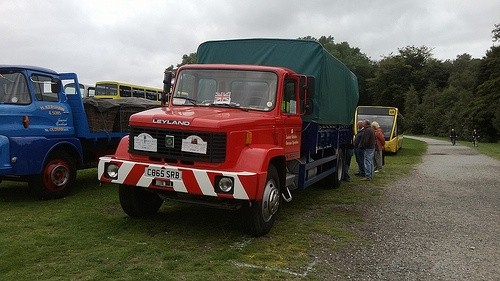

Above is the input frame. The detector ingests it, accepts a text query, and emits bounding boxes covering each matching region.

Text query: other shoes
[374,170,379,172]
[361,177,371,180]
[354,172,364,176]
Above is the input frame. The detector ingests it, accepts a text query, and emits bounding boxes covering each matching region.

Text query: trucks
[0,63,164,200]
[97,38,360,238]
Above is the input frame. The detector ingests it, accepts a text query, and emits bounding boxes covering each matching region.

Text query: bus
[353,105,405,154]
[95,81,189,103]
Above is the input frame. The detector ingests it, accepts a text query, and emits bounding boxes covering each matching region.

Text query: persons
[358,120,376,181]
[353,122,367,176]
[371,121,386,172]
[450,128,457,143]
[472,128,480,146]
